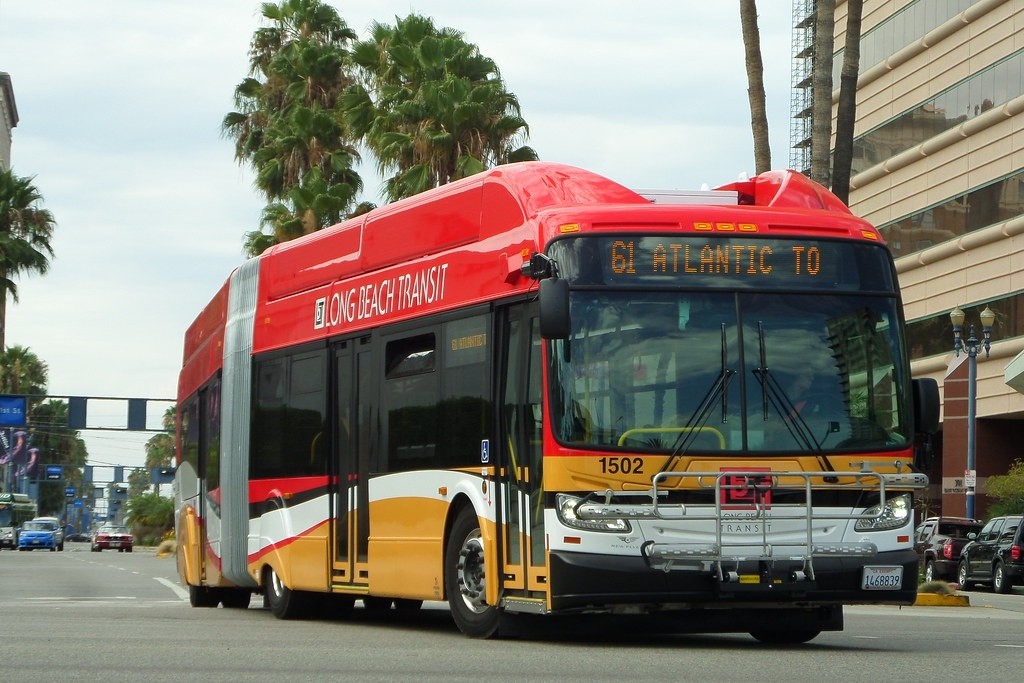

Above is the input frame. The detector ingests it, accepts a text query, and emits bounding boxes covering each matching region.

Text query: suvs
[956,515,1024,593]
[32,517,65,551]
[913,516,983,584]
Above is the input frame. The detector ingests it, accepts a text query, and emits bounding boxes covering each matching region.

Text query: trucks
[0,492,37,550]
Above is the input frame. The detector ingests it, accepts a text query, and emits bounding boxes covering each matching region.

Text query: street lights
[950,302,996,520]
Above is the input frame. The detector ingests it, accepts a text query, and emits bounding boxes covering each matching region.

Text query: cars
[17,520,58,553]
[91,525,133,552]
[64,532,92,542]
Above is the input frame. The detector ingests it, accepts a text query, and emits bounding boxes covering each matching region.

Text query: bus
[171,160,945,647]
